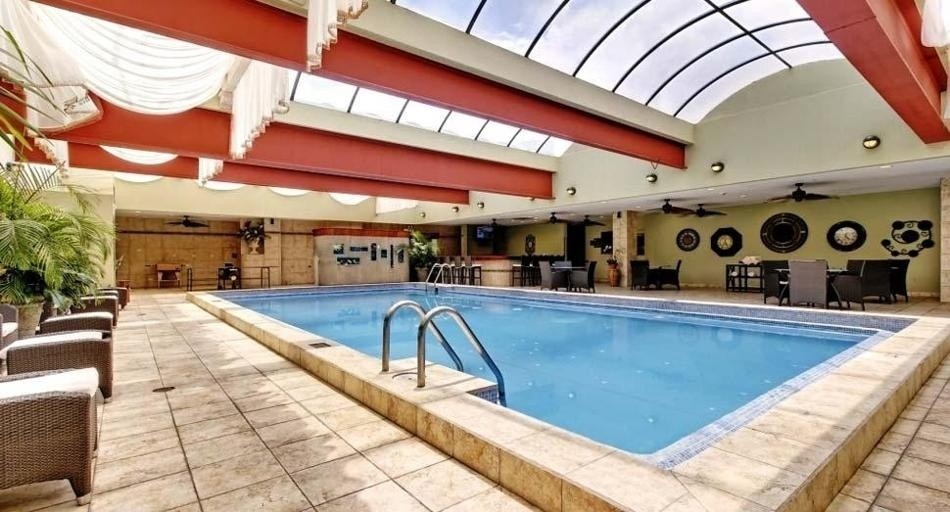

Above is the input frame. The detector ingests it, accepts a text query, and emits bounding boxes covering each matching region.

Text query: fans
[164,215,211,229]
[765,183,840,203]
[480,220,513,232]
[535,212,576,225]
[686,203,727,217]
[572,214,606,226]
[642,198,692,214]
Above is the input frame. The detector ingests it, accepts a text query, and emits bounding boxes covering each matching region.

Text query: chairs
[763,259,911,311]
[660,258,682,290]
[427,254,596,292]
[630,258,650,290]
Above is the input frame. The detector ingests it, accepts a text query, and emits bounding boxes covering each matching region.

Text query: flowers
[234,219,271,242]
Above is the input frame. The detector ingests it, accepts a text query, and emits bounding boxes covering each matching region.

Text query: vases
[249,240,259,254]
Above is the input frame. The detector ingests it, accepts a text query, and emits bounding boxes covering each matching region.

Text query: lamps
[567,187,576,195]
[646,174,657,183]
[451,206,459,212]
[419,212,425,218]
[477,202,485,208]
[862,134,880,150]
[711,162,723,172]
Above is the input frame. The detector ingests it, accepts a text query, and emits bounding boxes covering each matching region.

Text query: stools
[1,282,129,506]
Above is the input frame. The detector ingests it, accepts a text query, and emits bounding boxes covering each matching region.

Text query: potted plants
[395,228,438,282]
[0,151,114,337]
[606,258,617,268]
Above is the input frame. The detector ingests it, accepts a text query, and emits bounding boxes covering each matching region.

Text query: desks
[645,267,663,290]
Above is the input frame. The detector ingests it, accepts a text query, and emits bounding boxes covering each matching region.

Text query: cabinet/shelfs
[725,264,765,293]
